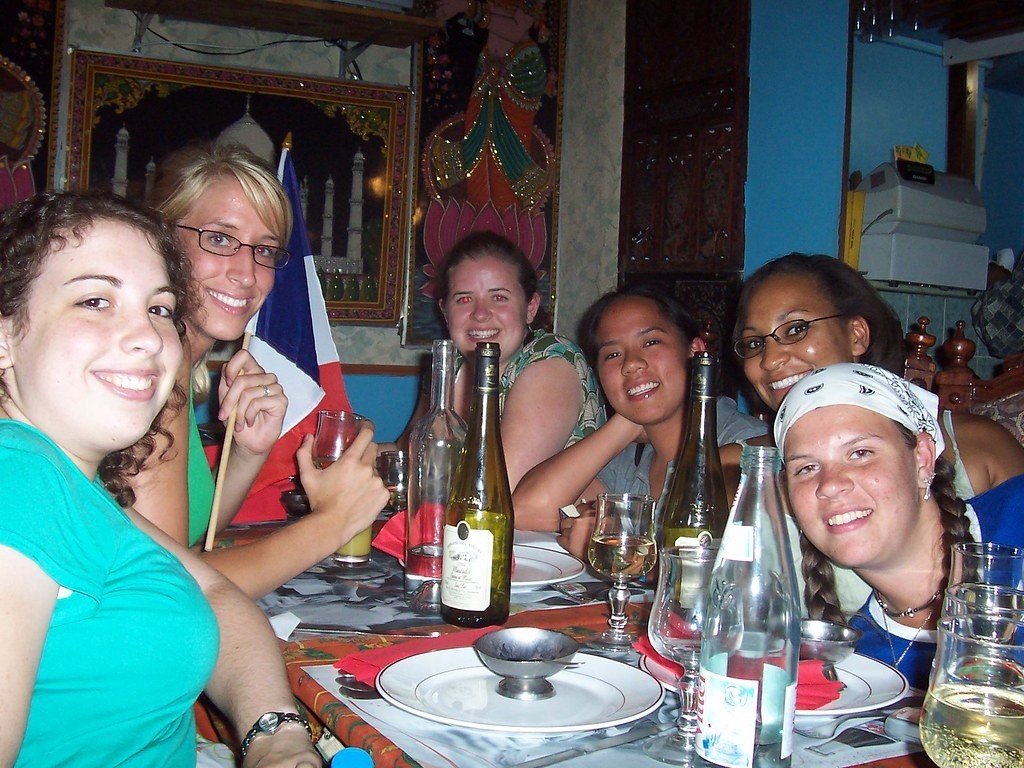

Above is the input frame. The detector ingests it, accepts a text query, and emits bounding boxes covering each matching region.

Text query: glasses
[733,312,856,359]
[176,224,293,270]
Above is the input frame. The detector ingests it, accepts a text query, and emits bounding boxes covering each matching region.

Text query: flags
[223,150,359,529]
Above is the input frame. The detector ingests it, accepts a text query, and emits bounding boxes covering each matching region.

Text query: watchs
[239,713,314,764]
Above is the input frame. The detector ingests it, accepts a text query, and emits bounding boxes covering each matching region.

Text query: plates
[399,544,585,592]
[639,651,909,721]
[375,646,665,732]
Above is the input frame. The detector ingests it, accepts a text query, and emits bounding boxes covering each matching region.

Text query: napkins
[627,612,841,710]
[371,502,516,580]
[332,625,506,689]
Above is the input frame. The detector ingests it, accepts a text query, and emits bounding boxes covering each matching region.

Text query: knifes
[508,721,679,768]
[295,622,440,637]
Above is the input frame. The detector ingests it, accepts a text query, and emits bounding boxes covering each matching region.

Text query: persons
[379,229,611,508]
[105,147,390,604]
[0,188,323,768]
[707,252,1023,630]
[511,287,770,586]
[771,359,1024,701]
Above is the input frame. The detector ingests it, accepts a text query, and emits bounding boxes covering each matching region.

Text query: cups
[382,450,408,512]
[312,409,370,564]
[919,542,1024,768]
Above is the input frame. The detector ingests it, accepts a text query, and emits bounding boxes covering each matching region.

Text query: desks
[189,514,939,768]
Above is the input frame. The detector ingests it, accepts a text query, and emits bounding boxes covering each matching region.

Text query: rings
[258,385,271,396]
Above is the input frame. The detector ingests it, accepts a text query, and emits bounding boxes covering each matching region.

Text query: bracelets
[640,576,647,582]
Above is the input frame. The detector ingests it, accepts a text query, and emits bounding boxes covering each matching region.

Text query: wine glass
[585,493,658,652]
[648,546,744,768]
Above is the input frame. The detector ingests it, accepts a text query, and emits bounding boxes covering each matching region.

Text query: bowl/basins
[799,618,863,680]
[279,490,310,515]
[474,627,579,701]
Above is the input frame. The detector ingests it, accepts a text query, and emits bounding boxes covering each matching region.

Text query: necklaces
[879,593,940,669]
[871,574,945,616]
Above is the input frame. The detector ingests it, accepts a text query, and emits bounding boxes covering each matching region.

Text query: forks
[794,709,901,739]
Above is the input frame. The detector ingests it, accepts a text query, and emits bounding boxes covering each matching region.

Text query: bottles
[803,718,903,757]
[405,340,514,628]
[696,445,801,768]
[662,351,730,622]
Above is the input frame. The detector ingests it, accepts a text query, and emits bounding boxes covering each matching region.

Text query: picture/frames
[61,46,417,327]
